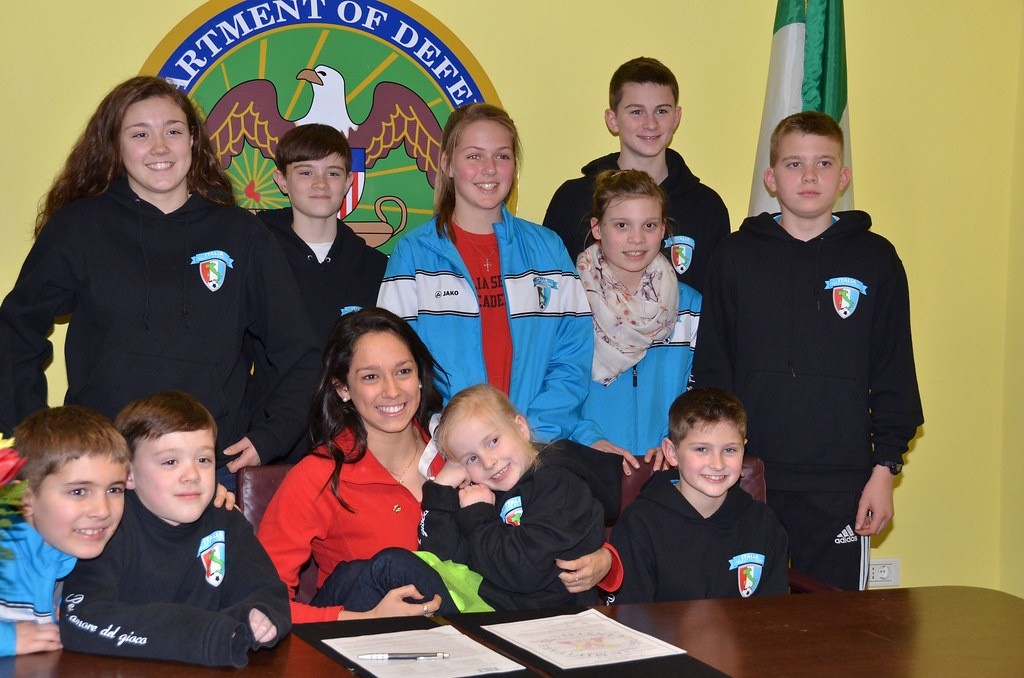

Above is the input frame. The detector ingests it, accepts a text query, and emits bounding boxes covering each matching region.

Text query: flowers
[0,432,32,557]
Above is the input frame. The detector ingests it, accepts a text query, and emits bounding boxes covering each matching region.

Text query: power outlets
[867,559,900,587]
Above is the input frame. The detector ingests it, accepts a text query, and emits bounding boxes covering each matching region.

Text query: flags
[750,0,854,213]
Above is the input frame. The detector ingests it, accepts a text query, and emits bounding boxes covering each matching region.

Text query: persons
[244,125,389,463]
[543,57,731,294]
[601,388,788,603]
[567,169,700,476]
[688,110,923,591]
[256,308,625,624]
[0,75,323,489]
[311,385,605,615]
[0,404,131,658]
[58,394,292,667]
[375,103,595,444]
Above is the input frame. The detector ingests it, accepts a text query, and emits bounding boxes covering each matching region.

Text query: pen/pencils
[358,652,451,661]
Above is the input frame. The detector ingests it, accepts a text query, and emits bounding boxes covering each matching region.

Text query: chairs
[596,454,841,606]
[237,464,296,530]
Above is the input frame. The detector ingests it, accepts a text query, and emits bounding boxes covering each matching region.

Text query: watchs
[879,461,902,473]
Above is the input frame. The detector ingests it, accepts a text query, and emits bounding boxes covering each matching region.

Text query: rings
[576,573,579,581]
[423,603,429,615]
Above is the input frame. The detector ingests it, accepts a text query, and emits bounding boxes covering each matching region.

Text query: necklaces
[390,444,419,484]
[461,224,496,271]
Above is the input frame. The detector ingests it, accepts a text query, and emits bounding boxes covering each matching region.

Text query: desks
[0,586,1024,678]
